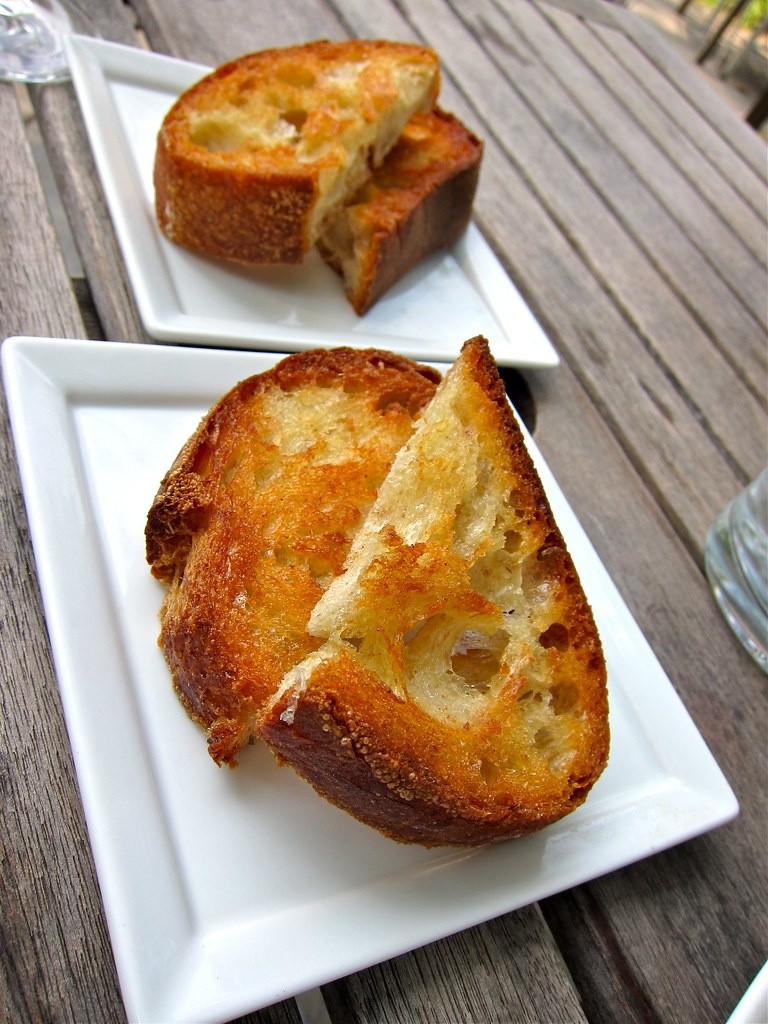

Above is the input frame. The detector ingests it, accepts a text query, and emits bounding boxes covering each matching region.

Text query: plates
[0,31,767,1024]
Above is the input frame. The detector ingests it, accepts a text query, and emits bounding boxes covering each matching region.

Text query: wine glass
[0,0,73,83]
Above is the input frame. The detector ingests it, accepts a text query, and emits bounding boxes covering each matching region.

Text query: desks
[0,0,767,1024]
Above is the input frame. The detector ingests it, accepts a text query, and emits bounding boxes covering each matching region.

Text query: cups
[702,470,768,676]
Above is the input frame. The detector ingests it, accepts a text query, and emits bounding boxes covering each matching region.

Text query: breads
[254,335,610,847]
[313,105,483,318]
[151,38,443,267]
[144,344,446,768]
